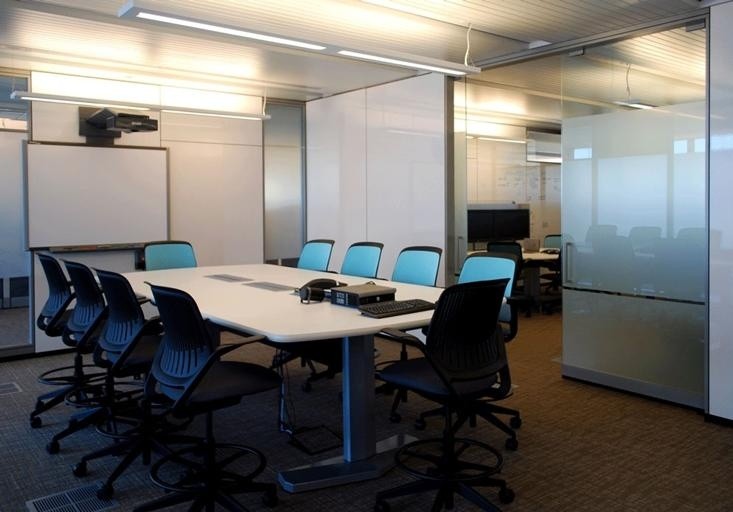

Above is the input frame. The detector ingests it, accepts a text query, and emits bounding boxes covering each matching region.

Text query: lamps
[117,0,482,77]
[467,118,527,144]
[9,71,271,121]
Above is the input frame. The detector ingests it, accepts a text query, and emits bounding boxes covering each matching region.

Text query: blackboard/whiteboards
[18,139,172,252]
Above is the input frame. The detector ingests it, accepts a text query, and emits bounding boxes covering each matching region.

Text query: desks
[95,263,511,493]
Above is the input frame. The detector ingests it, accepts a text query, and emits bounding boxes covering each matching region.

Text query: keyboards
[357,298,435,318]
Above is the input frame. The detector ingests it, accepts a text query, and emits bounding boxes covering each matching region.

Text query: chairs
[586,225,722,300]
[33,251,124,425]
[135,281,287,510]
[73,266,206,500]
[141,241,198,268]
[389,252,522,451]
[302,243,382,396]
[372,277,516,512]
[466,235,561,317]
[33,258,158,455]
[378,245,443,401]
[272,239,334,370]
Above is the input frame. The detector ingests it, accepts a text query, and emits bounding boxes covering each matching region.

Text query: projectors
[104,114,159,133]
